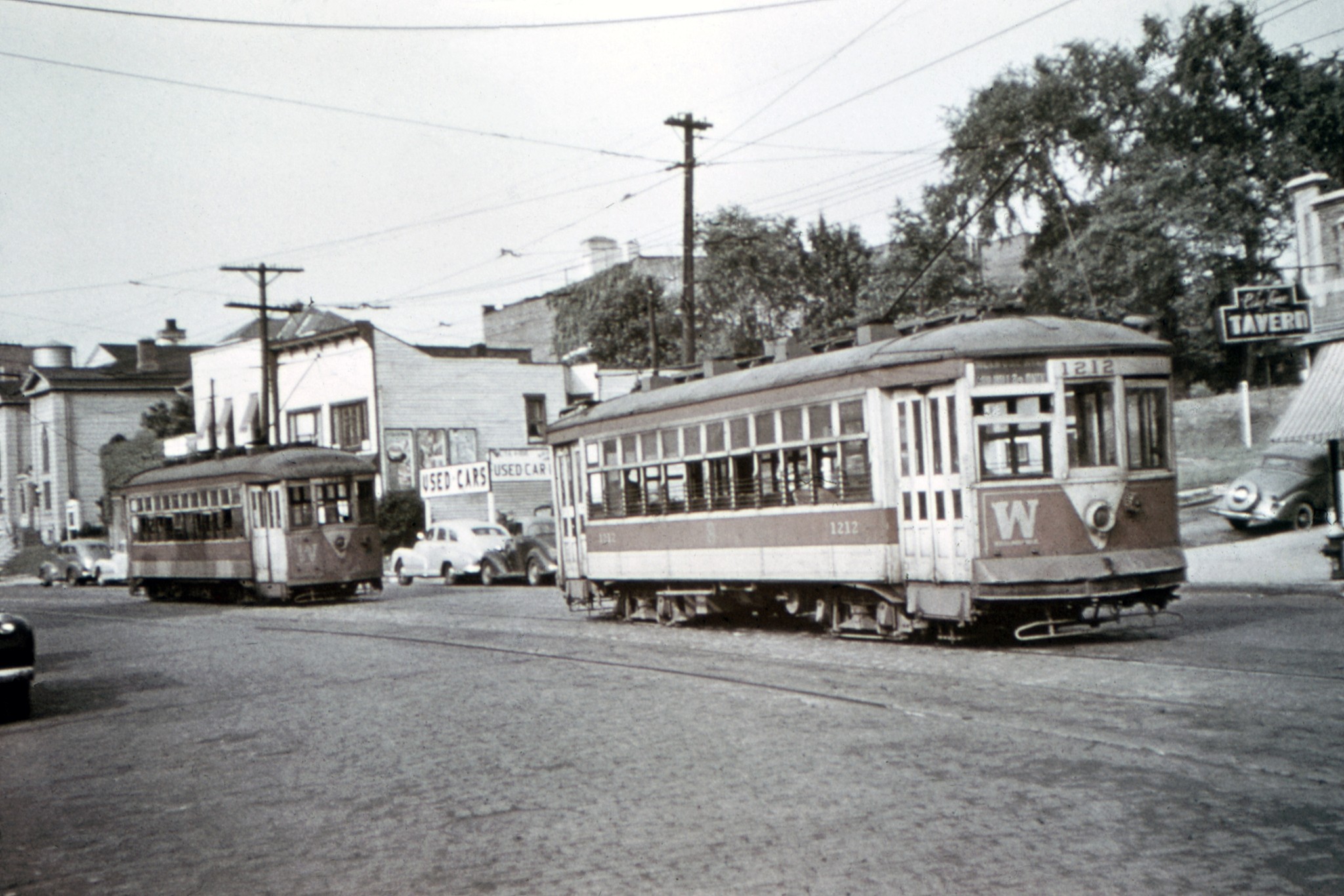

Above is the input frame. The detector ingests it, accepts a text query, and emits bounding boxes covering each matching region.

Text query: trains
[541,316,1186,648]
[125,440,385,608]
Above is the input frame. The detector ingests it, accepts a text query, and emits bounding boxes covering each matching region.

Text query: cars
[91,537,131,586]
[392,518,513,582]
[1207,440,1339,534]
[481,518,563,588]
[498,510,536,538]
[0,612,35,725]
[37,537,111,588]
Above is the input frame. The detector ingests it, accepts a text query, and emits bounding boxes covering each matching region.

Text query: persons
[606,467,642,518]
